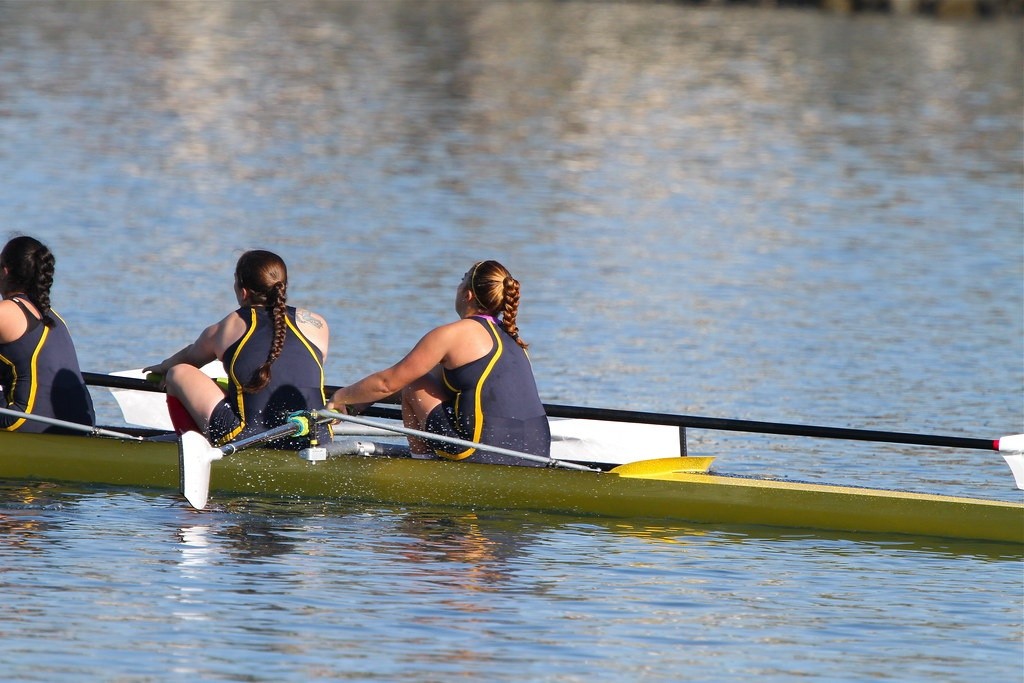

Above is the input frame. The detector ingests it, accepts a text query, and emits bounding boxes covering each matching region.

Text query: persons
[323,260,551,469]
[142,251,333,452]
[0,236,95,437]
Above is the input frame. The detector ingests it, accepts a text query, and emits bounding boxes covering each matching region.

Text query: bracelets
[161,384,167,392]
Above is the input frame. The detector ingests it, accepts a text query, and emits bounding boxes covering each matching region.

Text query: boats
[0,424,1024,547]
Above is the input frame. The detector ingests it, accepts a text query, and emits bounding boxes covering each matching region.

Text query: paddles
[362,402,1024,492]
[321,409,717,478]
[329,417,688,463]
[145,372,403,405]
[175,405,355,510]
[83,359,230,433]
[80,370,168,393]
